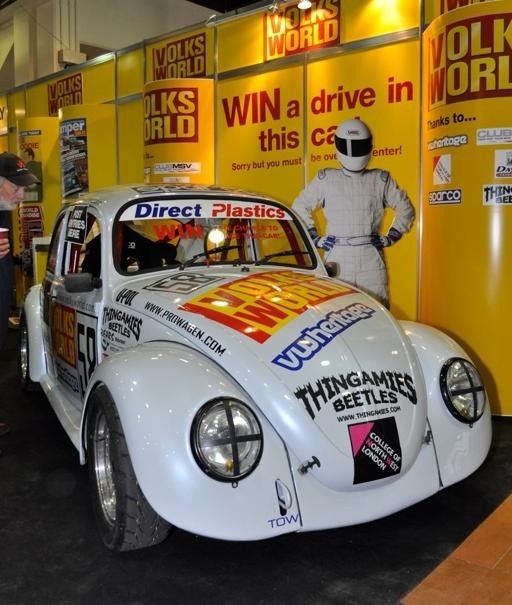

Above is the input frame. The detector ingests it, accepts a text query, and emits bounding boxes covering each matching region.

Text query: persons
[23,148,42,179]
[292,119,416,312]
[0,151,41,260]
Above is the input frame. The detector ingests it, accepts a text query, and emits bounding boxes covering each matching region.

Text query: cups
[0,228,9,241]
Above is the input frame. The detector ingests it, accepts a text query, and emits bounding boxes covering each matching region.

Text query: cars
[16,182,496,555]
[63,160,84,200]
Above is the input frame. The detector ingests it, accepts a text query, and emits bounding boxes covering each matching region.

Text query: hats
[1,151,41,186]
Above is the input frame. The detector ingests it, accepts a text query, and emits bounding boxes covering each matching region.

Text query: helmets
[334,119,372,172]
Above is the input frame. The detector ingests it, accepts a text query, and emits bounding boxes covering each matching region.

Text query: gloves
[371,235,392,249]
[315,235,336,251]
